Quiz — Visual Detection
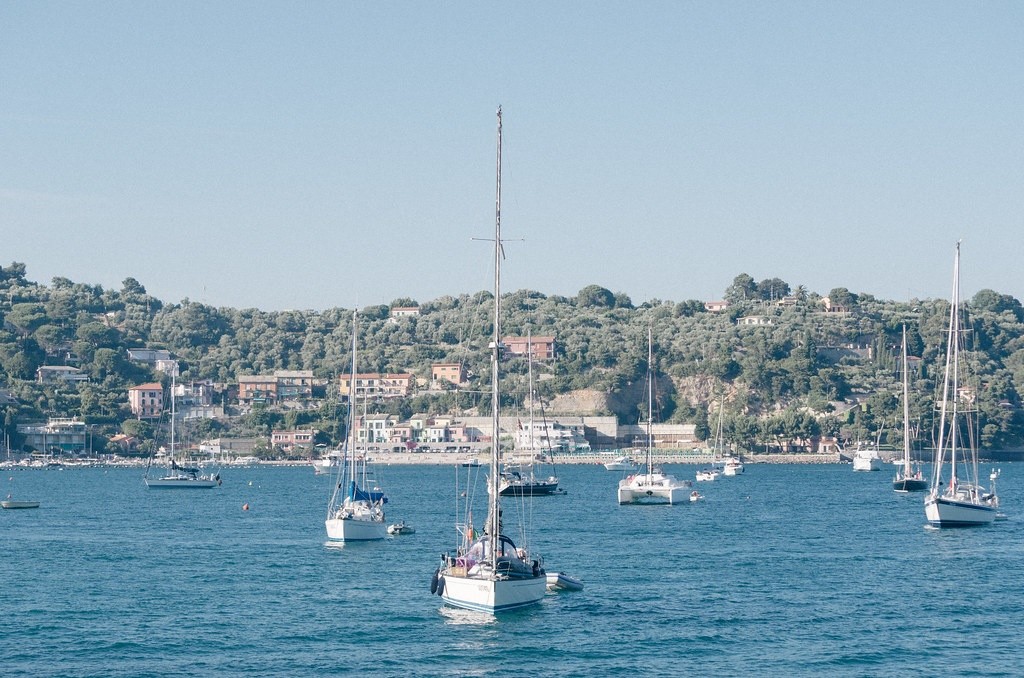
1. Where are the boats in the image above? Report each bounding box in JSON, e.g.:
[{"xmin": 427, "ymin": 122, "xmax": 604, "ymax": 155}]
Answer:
[
  {"xmin": 1, "ymin": 499, "xmax": 41, "ymax": 510},
  {"xmin": 546, "ymin": 570, "xmax": 585, "ymax": 594},
  {"xmin": 695, "ymin": 471, "xmax": 723, "ymax": 483},
  {"xmin": 724, "ymin": 455, "xmax": 744, "ymax": 477},
  {"xmin": 603, "ymin": 455, "xmax": 639, "ymax": 472},
  {"xmin": 852, "ymin": 449, "xmax": 884, "ymax": 472}
]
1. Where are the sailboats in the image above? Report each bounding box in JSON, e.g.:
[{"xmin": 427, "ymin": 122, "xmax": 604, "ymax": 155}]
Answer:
[
  {"xmin": 890, "ymin": 324, "xmax": 927, "ymax": 491},
  {"xmin": 312, "ymin": 307, "xmax": 419, "ymax": 542},
  {"xmin": 428, "ymin": 105, "xmax": 549, "ymax": 610},
  {"xmin": 923, "ymin": 239, "xmax": 998, "ymax": 526},
  {"xmin": 616, "ymin": 324, "xmax": 694, "ymax": 505},
  {"xmin": 498, "ymin": 322, "xmax": 560, "ymax": 497},
  {"xmin": 142, "ymin": 365, "xmax": 222, "ymax": 489}
]
